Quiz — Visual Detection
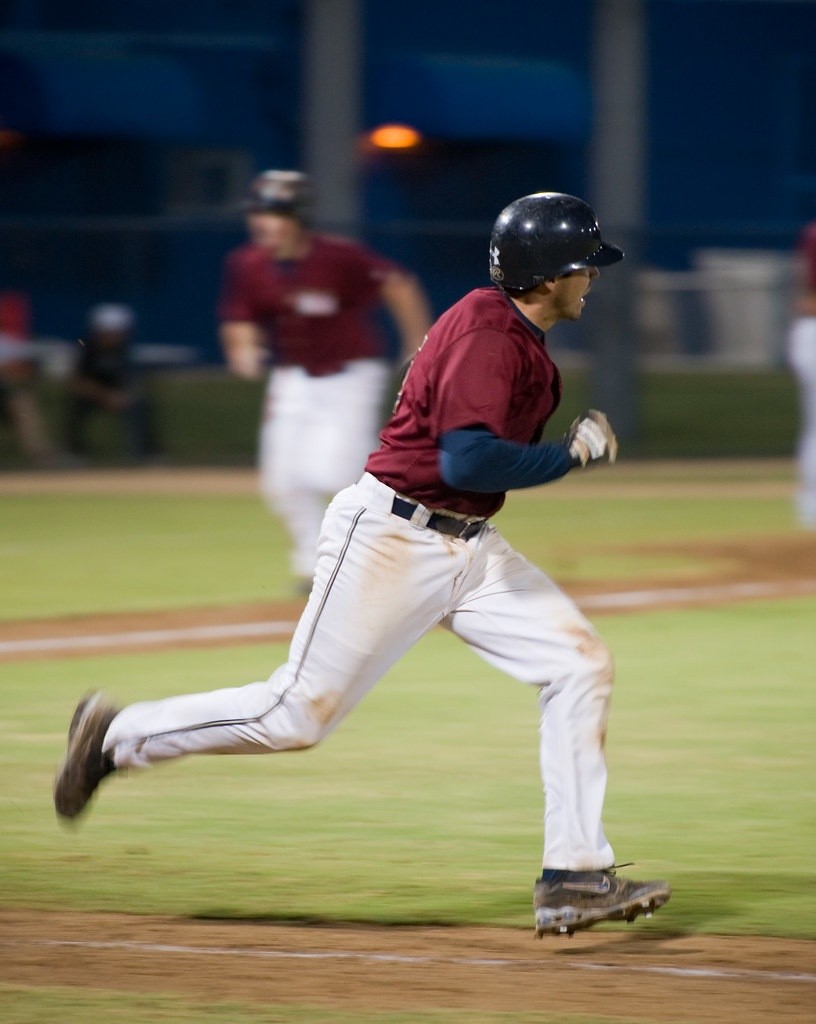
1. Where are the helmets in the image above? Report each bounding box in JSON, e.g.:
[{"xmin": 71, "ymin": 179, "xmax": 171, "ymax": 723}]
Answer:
[
  {"xmin": 231, "ymin": 170, "xmax": 322, "ymax": 226},
  {"xmin": 489, "ymin": 192, "xmax": 624, "ymax": 290}
]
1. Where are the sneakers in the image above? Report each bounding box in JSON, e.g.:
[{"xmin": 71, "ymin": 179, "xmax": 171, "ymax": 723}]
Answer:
[
  {"xmin": 54, "ymin": 689, "xmax": 120, "ymax": 814},
  {"xmin": 533, "ymin": 863, "xmax": 671, "ymax": 939}
]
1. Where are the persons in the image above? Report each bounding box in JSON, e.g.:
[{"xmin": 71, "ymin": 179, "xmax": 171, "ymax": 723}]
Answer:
[
  {"xmin": 58, "ymin": 302, "xmax": 158, "ymax": 464},
  {"xmin": 787, "ymin": 223, "xmax": 816, "ymax": 536},
  {"xmin": 220, "ymin": 170, "xmax": 428, "ymax": 598},
  {"xmin": 55, "ymin": 192, "xmax": 670, "ymax": 942},
  {"xmin": 0, "ymin": 293, "xmax": 56, "ymax": 467}
]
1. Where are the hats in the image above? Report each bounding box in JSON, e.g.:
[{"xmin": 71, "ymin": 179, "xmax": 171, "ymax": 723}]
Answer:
[{"xmin": 90, "ymin": 301, "xmax": 134, "ymax": 331}]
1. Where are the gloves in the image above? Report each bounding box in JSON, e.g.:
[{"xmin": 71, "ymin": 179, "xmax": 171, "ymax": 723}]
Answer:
[{"xmin": 563, "ymin": 409, "xmax": 619, "ymax": 469}]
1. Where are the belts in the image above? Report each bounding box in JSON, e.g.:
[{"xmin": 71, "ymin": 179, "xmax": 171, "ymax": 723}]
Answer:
[{"xmin": 391, "ymin": 496, "xmax": 489, "ymax": 539}]
